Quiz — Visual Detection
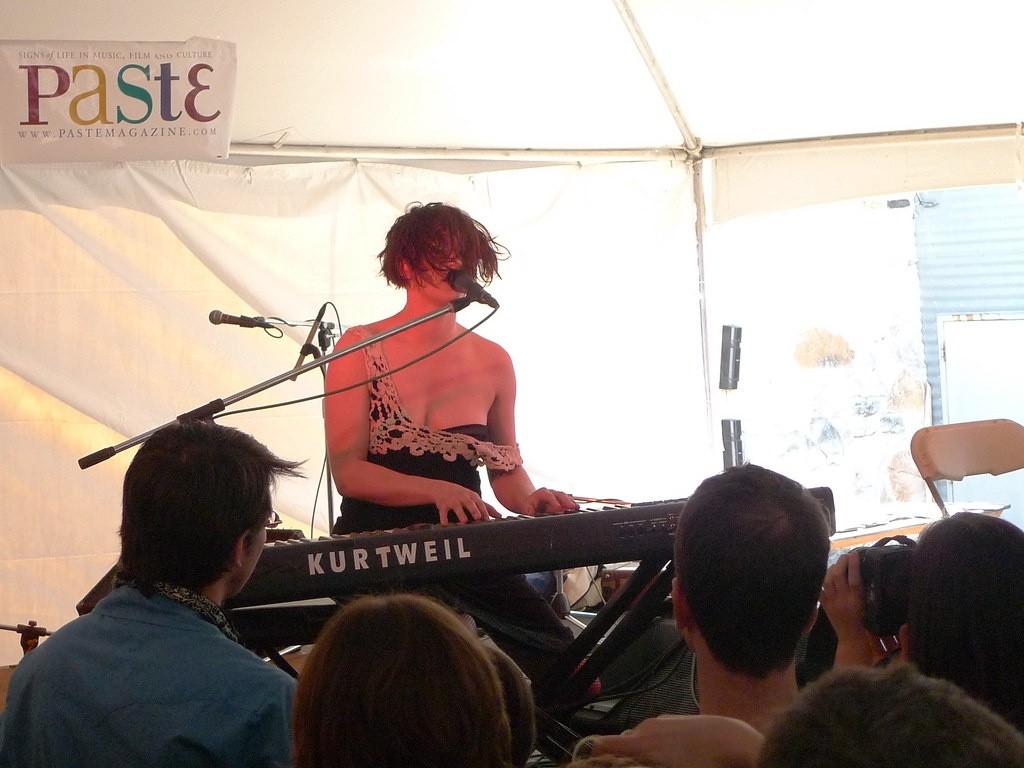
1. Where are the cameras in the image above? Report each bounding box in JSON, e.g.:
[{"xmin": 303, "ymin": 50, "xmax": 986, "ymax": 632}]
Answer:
[{"xmin": 847, "ymin": 545, "xmax": 915, "ymax": 637}]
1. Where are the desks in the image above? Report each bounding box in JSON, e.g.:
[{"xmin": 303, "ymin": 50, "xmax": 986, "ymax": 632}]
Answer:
[{"xmin": 830, "ymin": 501, "xmax": 1007, "ymax": 555}]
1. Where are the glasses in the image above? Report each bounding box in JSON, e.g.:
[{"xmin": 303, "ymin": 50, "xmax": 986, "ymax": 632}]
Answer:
[{"xmin": 260, "ymin": 511, "xmax": 282, "ymax": 528}]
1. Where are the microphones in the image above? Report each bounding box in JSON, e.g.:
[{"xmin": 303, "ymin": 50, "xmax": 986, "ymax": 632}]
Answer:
[
  {"xmin": 209, "ymin": 310, "xmax": 273, "ymax": 328},
  {"xmin": 291, "ymin": 304, "xmax": 327, "ymax": 381},
  {"xmin": 447, "ymin": 269, "xmax": 499, "ymax": 309}
]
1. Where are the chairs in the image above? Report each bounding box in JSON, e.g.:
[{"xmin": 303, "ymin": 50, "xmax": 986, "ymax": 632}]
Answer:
[{"xmin": 911, "ymin": 419, "xmax": 1024, "ymax": 518}]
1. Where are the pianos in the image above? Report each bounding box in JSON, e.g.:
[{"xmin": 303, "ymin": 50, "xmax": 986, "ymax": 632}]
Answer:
[{"xmin": 220, "ymin": 484, "xmax": 841, "ymax": 610}]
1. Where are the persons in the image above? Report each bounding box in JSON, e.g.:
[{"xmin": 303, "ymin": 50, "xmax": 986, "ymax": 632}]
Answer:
[
  {"xmin": 7, "ymin": 421, "xmax": 1024, "ymax": 767},
  {"xmin": 324, "ymin": 203, "xmax": 577, "ymax": 699}
]
